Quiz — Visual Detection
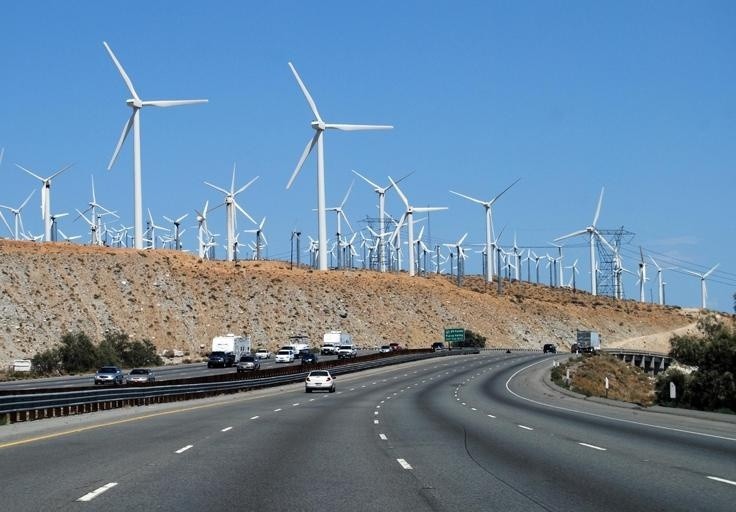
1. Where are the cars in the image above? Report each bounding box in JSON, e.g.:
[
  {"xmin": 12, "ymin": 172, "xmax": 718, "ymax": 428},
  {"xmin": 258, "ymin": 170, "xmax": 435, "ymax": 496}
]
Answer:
[
  {"xmin": 304, "ymin": 370, "xmax": 336, "ymax": 394},
  {"xmin": 125, "ymin": 368, "xmax": 154, "ymax": 384},
  {"xmin": 207, "ymin": 331, "xmax": 356, "ymax": 373},
  {"xmin": 543, "ymin": 344, "xmax": 556, "ymax": 353},
  {"xmin": 93, "ymin": 366, "xmax": 123, "ymax": 385},
  {"xmin": 431, "ymin": 342, "xmax": 443, "ymax": 351},
  {"xmin": 571, "ymin": 344, "xmax": 576, "ymax": 353},
  {"xmin": 379, "ymin": 343, "xmax": 401, "ymax": 354}
]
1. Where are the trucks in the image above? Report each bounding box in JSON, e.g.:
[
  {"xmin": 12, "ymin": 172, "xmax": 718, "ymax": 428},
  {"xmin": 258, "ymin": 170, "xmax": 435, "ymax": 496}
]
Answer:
[{"xmin": 576, "ymin": 330, "xmax": 601, "ymax": 352}]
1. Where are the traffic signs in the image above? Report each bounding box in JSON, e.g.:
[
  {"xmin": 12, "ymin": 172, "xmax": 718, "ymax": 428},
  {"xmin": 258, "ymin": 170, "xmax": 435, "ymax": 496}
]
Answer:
[{"xmin": 444, "ymin": 329, "xmax": 465, "ymax": 342}]
